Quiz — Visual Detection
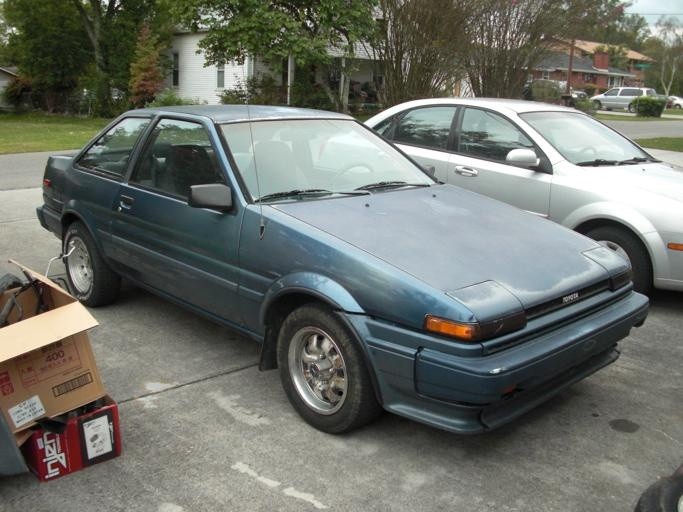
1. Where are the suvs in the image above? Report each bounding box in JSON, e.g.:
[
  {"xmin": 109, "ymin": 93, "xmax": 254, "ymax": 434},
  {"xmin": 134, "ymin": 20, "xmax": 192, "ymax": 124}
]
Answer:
[{"xmin": 522, "ymin": 77, "xmax": 587, "ymax": 105}]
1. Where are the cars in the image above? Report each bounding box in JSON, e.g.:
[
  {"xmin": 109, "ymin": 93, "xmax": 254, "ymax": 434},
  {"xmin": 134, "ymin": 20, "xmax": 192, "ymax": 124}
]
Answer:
[
  {"xmin": 111, "ymin": 88, "xmax": 123, "ymax": 103},
  {"xmin": 36, "ymin": 101, "xmax": 649, "ymax": 436},
  {"xmin": 316, "ymin": 95, "xmax": 682, "ymax": 293},
  {"xmin": 666, "ymin": 93, "xmax": 682, "ymax": 109}
]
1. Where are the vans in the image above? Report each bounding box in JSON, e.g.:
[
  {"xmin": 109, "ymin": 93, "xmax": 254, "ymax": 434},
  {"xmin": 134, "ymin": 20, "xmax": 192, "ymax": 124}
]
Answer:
[{"xmin": 587, "ymin": 86, "xmax": 657, "ymax": 113}]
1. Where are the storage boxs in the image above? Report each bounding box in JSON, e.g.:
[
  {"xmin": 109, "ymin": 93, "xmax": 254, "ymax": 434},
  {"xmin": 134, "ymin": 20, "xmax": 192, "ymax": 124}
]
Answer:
[
  {"xmin": 16, "ymin": 394, "xmax": 124, "ymax": 481},
  {"xmin": 0, "ymin": 256, "xmax": 107, "ymax": 439}
]
